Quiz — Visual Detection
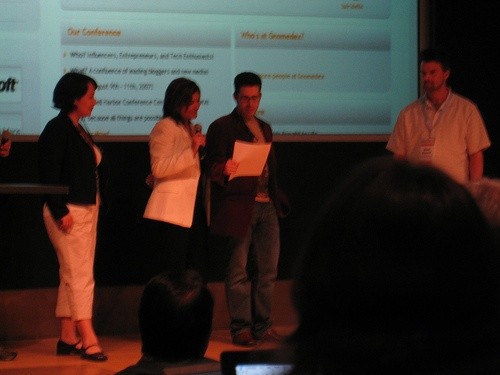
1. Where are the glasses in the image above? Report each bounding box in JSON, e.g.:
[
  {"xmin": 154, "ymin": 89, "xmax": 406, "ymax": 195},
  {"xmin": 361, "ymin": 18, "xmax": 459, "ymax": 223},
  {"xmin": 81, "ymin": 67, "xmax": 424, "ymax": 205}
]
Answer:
[{"xmin": 237, "ymin": 93, "xmax": 261, "ymax": 102}]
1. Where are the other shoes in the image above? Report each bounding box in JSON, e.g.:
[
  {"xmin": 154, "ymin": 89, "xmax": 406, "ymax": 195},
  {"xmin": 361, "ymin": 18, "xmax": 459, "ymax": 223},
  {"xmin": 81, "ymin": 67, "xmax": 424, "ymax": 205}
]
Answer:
[
  {"xmin": 57, "ymin": 338, "xmax": 83, "ymax": 356},
  {"xmin": 232, "ymin": 330, "xmax": 256, "ymax": 347},
  {"xmin": 253, "ymin": 328, "xmax": 284, "ymax": 343},
  {"xmin": 80, "ymin": 344, "xmax": 108, "ymax": 362}
]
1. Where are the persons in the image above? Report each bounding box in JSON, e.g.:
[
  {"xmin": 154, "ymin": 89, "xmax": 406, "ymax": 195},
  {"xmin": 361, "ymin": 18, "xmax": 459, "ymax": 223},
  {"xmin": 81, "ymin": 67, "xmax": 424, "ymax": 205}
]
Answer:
[
  {"xmin": 388, "ymin": 51, "xmax": 492, "ymax": 181},
  {"xmin": 0, "ymin": 127, "xmax": 20, "ymax": 361},
  {"xmin": 206, "ymin": 73, "xmax": 287, "ymax": 348},
  {"xmin": 288, "ymin": 156, "xmax": 500, "ymax": 374},
  {"xmin": 143, "ymin": 77, "xmax": 207, "ymax": 281},
  {"xmin": 38, "ymin": 73, "xmax": 108, "ymax": 362},
  {"xmin": 115, "ymin": 270, "xmax": 221, "ymax": 375}
]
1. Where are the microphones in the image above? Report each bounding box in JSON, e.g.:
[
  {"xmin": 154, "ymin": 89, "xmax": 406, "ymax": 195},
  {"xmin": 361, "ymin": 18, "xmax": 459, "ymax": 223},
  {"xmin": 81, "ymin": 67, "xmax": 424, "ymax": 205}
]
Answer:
[{"xmin": 193, "ymin": 123, "xmax": 204, "ymax": 160}]
